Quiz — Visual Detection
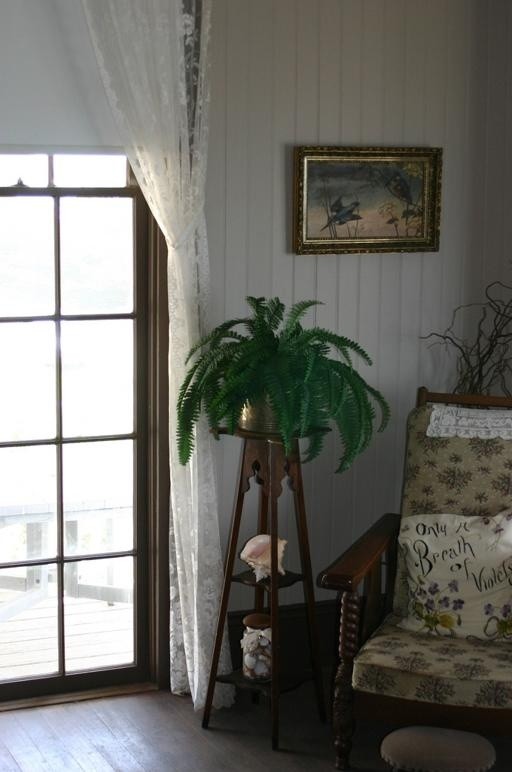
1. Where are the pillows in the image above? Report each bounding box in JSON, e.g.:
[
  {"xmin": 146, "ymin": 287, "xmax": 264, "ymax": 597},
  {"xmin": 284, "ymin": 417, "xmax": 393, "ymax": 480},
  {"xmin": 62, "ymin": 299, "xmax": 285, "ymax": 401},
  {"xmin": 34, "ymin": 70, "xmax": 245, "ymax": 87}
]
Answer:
[{"xmin": 397, "ymin": 512, "xmax": 511, "ymax": 637}]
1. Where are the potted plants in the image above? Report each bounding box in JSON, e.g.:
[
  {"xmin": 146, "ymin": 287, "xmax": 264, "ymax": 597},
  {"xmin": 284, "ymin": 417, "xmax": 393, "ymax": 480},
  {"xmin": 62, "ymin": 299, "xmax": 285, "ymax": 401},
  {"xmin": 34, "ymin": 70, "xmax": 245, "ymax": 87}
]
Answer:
[{"xmin": 177, "ymin": 295, "xmax": 390, "ymax": 475}]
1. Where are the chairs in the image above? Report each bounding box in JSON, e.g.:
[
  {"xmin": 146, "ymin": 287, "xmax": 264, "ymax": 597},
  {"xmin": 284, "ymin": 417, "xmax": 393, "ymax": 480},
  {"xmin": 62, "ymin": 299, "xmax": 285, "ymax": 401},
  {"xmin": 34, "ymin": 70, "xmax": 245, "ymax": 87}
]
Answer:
[{"xmin": 315, "ymin": 386, "xmax": 512, "ymax": 772}]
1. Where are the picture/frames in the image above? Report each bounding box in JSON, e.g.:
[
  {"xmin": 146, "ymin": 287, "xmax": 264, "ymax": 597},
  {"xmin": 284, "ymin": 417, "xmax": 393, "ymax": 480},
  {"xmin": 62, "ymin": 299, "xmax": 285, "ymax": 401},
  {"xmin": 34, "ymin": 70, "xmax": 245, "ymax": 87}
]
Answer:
[{"xmin": 292, "ymin": 142, "xmax": 442, "ymax": 256}]
